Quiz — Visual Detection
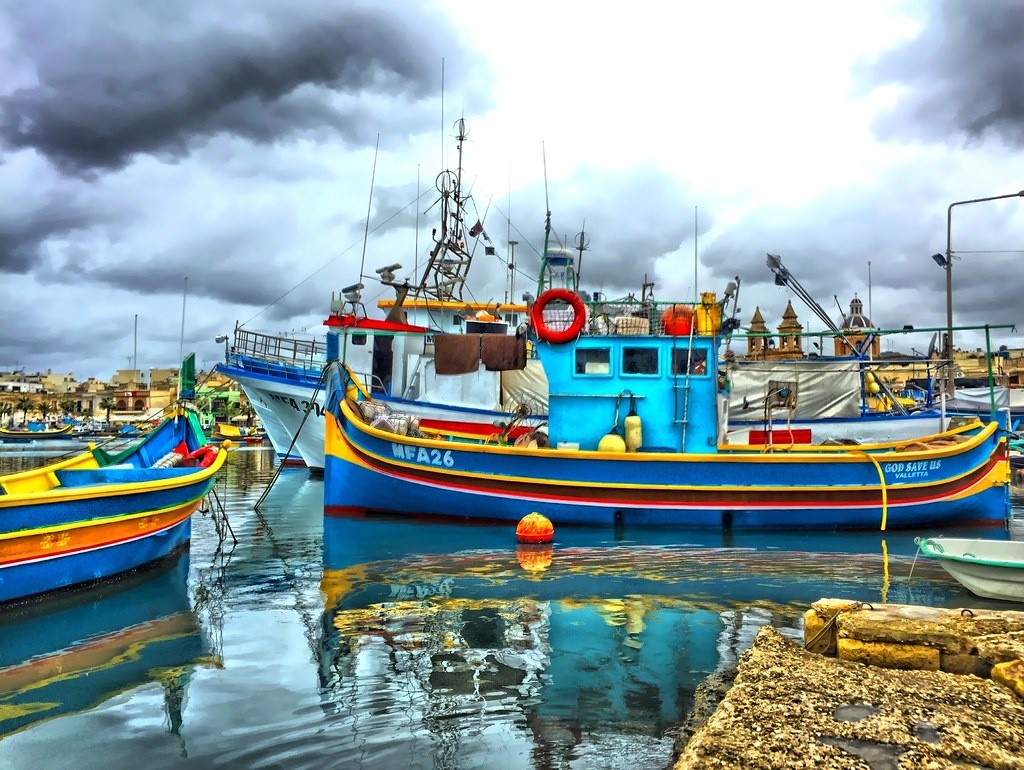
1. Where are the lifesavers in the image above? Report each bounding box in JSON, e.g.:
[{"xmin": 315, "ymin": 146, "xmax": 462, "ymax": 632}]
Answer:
[
  {"xmin": 531, "ymin": 289, "xmax": 585, "ymax": 343},
  {"xmin": 188, "ymin": 445, "xmax": 219, "ymax": 468}
]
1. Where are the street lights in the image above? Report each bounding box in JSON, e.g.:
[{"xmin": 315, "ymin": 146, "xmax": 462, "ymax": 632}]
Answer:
[{"xmin": 931, "ymin": 252, "xmax": 955, "ymax": 400}]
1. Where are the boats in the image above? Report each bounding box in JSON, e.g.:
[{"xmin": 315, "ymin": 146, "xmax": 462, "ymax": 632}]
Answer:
[
  {"xmin": 0, "ymin": 351, "xmax": 233, "ymax": 603},
  {"xmin": 79, "ymin": 436, "xmax": 117, "ymax": 442},
  {"xmin": 0, "ymin": 423, "xmax": 74, "ymax": 439},
  {"xmin": 323, "ymin": 116, "xmax": 1012, "ymax": 525},
  {"xmin": 213, "ymin": 55, "xmax": 524, "ymax": 481}
]
